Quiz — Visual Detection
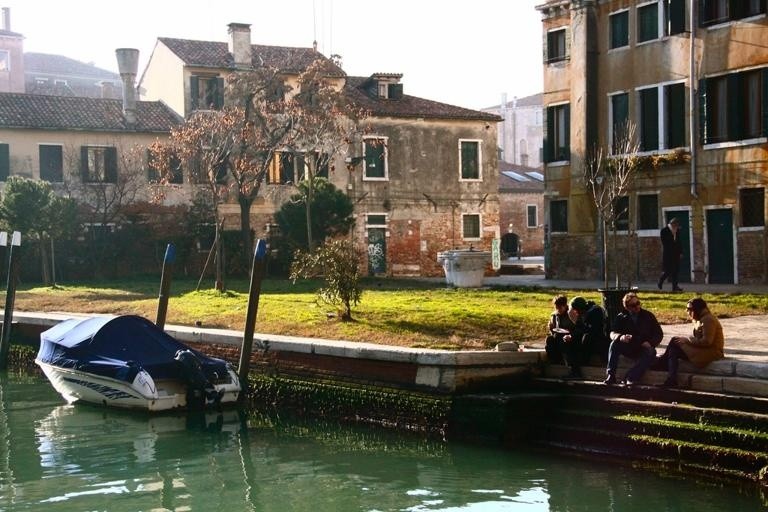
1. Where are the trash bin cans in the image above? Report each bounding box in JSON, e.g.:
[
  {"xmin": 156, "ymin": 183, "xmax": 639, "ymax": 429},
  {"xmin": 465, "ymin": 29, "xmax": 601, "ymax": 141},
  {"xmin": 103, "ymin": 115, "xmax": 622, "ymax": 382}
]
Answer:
[{"xmin": 598, "ymin": 286, "xmax": 639, "ymax": 327}]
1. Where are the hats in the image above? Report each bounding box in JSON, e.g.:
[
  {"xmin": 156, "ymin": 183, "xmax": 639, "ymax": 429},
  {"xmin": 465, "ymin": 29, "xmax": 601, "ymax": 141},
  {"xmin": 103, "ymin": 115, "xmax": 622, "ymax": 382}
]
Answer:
[{"xmin": 570, "ymin": 296, "xmax": 586, "ymax": 310}]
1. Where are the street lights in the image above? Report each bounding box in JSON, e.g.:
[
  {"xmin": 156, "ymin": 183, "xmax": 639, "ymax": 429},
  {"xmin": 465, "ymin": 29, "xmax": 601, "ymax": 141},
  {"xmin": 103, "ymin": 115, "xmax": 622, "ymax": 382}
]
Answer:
[
  {"xmin": 595, "ymin": 176, "xmax": 606, "ymax": 282},
  {"xmin": 348, "ymin": 155, "xmax": 375, "ymax": 257}
]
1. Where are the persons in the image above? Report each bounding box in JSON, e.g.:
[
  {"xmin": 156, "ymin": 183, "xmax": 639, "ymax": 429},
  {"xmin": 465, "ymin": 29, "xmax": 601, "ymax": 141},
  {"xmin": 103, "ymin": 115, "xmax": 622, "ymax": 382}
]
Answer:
[
  {"xmin": 658, "ymin": 217, "xmax": 684, "ymax": 291},
  {"xmin": 545, "ymin": 293, "xmax": 724, "ymax": 388}
]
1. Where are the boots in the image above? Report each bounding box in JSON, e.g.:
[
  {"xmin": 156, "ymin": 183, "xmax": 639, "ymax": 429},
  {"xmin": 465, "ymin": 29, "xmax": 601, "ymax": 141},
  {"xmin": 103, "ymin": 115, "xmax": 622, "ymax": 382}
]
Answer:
[{"xmin": 655, "ymin": 378, "xmax": 678, "ymax": 389}]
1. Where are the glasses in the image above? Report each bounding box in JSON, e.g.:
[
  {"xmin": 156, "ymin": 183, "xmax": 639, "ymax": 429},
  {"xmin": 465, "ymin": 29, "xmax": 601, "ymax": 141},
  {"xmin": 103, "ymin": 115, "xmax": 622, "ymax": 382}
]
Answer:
[
  {"xmin": 627, "ymin": 301, "xmax": 640, "ymax": 307},
  {"xmin": 687, "ymin": 308, "xmax": 695, "ymax": 310}
]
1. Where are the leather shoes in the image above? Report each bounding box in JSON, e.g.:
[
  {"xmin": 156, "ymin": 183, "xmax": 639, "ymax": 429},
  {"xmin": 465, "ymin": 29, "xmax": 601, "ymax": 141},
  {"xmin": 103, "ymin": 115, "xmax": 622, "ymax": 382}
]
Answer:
[
  {"xmin": 621, "ymin": 377, "xmax": 634, "ymax": 385},
  {"xmin": 602, "ymin": 374, "xmax": 616, "ymax": 383}
]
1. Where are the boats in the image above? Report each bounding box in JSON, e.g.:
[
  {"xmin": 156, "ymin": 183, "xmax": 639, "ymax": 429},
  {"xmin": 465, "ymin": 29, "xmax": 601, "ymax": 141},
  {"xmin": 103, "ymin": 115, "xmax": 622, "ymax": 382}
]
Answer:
[
  {"xmin": 35, "ymin": 404, "xmax": 240, "ymax": 478},
  {"xmin": 34, "ymin": 315, "xmax": 241, "ymax": 413}
]
